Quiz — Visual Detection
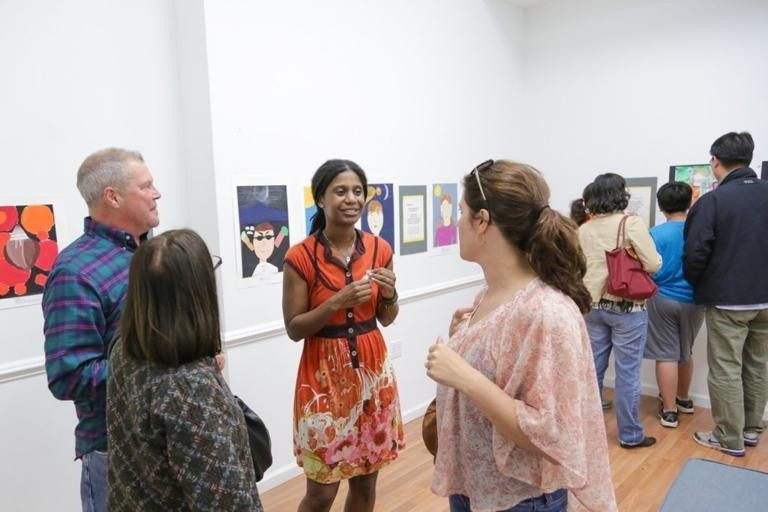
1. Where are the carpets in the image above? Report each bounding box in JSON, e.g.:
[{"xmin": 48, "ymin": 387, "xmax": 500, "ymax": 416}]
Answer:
[{"xmin": 656, "ymin": 457, "xmax": 767, "ymax": 512}]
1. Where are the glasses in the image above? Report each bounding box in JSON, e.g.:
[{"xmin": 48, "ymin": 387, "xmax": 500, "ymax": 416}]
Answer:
[
  {"xmin": 207, "ymin": 257, "xmax": 222, "ymax": 274},
  {"xmin": 473, "ymin": 159, "xmax": 493, "ymax": 209},
  {"xmin": 253, "ymin": 235, "xmax": 274, "ymax": 240}
]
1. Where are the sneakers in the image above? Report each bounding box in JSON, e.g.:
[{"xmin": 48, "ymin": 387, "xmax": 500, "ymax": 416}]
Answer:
[
  {"xmin": 658, "ymin": 393, "xmax": 694, "ymax": 414},
  {"xmin": 658, "ymin": 406, "xmax": 679, "ymax": 428},
  {"xmin": 601, "ymin": 399, "xmax": 612, "ymax": 409},
  {"xmin": 693, "ymin": 431, "xmax": 746, "ymax": 457},
  {"xmin": 744, "ymin": 431, "xmax": 762, "ymax": 447}
]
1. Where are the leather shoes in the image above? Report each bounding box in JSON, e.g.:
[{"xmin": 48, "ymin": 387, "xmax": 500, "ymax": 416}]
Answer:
[{"xmin": 620, "ymin": 436, "xmax": 655, "ymax": 449}]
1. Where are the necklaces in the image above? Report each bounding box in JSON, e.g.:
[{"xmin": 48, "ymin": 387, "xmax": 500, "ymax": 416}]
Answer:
[{"xmin": 322, "ymin": 231, "xmax": 357, "ymax": 270}]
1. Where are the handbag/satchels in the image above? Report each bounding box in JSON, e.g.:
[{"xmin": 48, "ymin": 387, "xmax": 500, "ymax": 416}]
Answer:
[
  {"xmin": 604, "ymin": 214, "xmax": 658, "ymax": 300},
  {"xmin": 234, "ymin": 395, "xmax": 272, "ymax": 483},
  {"xmin": 422, "ymin": 398, "xmax": 437, "ymax": 458}
]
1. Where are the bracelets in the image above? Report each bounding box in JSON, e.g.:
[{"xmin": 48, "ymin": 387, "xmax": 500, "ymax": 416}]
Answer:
[{"xmin": 378, "ymin": 291, "xmax": 397, "ymax": 310}]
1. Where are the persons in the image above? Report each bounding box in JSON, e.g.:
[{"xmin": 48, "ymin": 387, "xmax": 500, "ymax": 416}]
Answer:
[
  {"xmin": 570, "ymin": 183, "xmax": 598, "ymax": 225},
  {"xmin": 41, "ymin": 146, "xmax": 161, "ymax": 512},
  {"xmin": 424, "ymin": 159, "xmax": 617, "ymax": 512},
  {"xmin": 106, "ymin": 229, "xmax": 265, "ymax": 512},
  {"xmin": 435, "ymin": 194, "xmax": 457, "ymax": 248},
  {"xmin": 241, "ymin": 222, "xmax": 289, "ymax": 280},
  {"xmin": 682, "ymin": 132, "xmax": 768, "ymax": 457},
  {"xmin": 283, "ymin": 159, "xmax": 400, "ymax": 512},
  {"xmin": 644, "ymin": 180, "xmax": 705, "ymax": 429},
  {"xmin": 366, "ymin": 199, "xmax": 392, "ymax": 252},
  {"xmin": 575, "ymin": 174, "xmax": 657, "ymax": 449}
]
